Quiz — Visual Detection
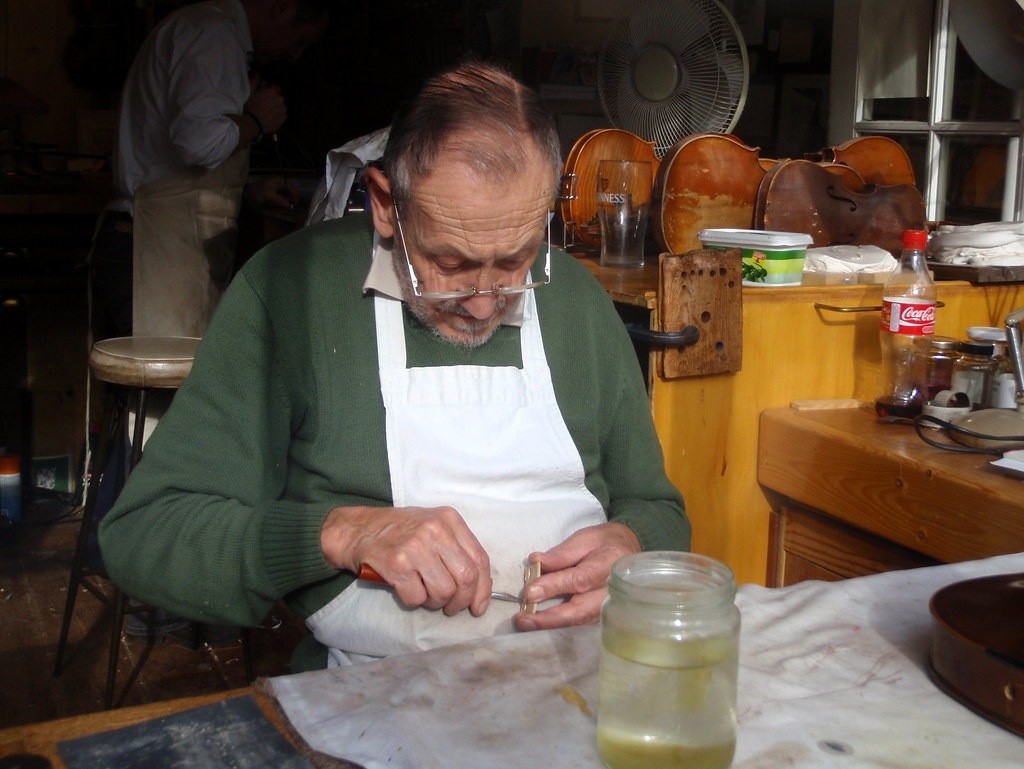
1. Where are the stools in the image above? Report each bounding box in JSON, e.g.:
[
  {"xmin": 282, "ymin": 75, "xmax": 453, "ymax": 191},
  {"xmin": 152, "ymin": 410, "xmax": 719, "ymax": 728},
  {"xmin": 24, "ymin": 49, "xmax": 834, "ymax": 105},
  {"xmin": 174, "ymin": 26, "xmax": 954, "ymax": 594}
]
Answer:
[{"xmin": 51, "ymin": 335, "xmax": 254, "ymax": 710}]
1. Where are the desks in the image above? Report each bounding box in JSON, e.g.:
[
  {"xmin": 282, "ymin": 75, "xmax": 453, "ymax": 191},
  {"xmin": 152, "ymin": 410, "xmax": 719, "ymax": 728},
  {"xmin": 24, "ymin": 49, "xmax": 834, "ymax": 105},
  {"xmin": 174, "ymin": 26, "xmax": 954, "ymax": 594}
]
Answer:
[
  {"xmin": 0, "ymin": 548, "xmax": 1023, "ymax": 769},
  {"xmin": 546, "ymin": 239, "xmax": 1023, "ymax": 588},
  {"xmin": 755, "ymin": 402, "xmax": 1024, "ymax": 591}
]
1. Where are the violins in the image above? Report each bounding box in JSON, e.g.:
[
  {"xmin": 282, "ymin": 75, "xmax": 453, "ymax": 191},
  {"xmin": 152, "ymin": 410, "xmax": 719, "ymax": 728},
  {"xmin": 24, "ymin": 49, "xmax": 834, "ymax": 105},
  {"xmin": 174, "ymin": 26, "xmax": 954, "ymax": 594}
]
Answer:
[
  {"xmin": 555, "ymin": 113, "xmax": 928, "ymax": 267},
  {"xmin": 920, "ymin": 570, "xmax": 1024, "ymax": 739}
]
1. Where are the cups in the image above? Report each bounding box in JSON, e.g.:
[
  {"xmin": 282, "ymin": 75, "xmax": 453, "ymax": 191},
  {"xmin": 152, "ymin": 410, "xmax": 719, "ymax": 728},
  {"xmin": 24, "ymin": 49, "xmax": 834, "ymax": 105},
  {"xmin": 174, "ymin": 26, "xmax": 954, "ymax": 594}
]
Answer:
[{"xmin": 596, "ymin": 159, "xmax": 654, "ymax": 269}]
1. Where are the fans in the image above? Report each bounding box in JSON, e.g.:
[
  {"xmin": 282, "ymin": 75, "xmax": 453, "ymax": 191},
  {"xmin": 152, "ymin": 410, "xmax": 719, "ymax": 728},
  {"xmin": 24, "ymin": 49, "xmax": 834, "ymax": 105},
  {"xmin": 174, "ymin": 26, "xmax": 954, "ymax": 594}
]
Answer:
[{"xmin": 595, "ymin": 0, "xmax": 750, "ymax": 159}]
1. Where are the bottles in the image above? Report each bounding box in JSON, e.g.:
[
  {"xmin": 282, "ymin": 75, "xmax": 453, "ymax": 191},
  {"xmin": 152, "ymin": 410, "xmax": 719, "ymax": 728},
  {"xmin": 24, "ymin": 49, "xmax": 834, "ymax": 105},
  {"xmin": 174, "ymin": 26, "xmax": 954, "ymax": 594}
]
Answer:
[
  {"xmin": 0, "ymin": 454, "xmax": 22, "ymax": 528},
  {"xmin": 926, "ymin": 326, "xmax": 1018, "ymax": 411},
  {"xmin": 595, "ymin": 550, "xmax": 741, "ymax": 769},
  {"xmin": 874, "ymin": 229, "xmax": 938, "ymax": 421}
]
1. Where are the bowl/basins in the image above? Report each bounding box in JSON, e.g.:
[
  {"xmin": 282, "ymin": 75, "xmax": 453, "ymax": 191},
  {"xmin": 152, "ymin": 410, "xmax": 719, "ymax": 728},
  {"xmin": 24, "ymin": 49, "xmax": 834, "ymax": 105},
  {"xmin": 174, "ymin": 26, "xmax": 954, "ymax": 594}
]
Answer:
[{"xmin": 698, "ymin": 228, "xmax": 815, "ymax": 287}]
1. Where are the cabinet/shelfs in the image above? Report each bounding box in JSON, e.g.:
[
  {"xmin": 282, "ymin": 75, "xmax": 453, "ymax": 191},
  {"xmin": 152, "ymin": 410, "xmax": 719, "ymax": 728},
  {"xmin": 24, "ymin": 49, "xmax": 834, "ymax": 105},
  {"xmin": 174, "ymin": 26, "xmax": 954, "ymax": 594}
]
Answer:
[{"xmin": 851, "ymin": 1, "xmax": 1024, "ymax": 233}]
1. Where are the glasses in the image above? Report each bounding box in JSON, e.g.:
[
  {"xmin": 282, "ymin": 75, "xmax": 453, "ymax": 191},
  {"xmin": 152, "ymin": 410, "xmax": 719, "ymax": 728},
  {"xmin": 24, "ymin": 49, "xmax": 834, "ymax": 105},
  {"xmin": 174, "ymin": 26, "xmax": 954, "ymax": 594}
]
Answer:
[{"xmin": 380, "ymin": 169, "xmax": 552, "ymax": 301}]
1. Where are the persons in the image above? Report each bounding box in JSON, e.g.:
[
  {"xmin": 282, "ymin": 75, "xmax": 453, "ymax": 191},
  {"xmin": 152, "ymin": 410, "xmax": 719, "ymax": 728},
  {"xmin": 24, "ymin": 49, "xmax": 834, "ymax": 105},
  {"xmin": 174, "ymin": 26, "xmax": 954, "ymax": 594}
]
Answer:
[
  {"xmin": 97, "ymin": 57, "xmax": 694, "ymax": 683},
  {"xmin": 88, "ymin": 0, "xmax": 332, "ymax": 635}
]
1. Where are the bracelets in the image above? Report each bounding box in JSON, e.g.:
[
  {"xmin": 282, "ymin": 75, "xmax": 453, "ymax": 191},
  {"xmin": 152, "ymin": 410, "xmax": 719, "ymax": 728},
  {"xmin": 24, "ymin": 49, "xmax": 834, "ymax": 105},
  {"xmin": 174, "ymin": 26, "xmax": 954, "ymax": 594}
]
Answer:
[{"xmin": 242, "ymin": 113, "xmax": 263, "ymax": 146}]
